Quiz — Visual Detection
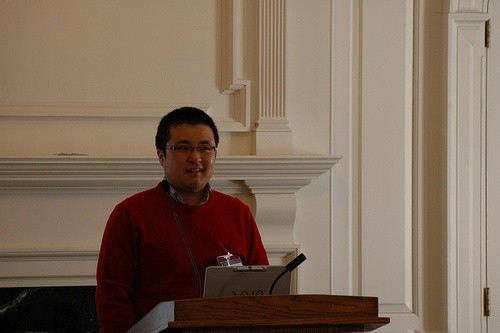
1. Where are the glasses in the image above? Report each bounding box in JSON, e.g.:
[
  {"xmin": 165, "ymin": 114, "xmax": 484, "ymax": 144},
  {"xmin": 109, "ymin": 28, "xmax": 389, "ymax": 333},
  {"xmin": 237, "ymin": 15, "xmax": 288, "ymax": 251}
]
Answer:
[{"xmin": 164, "ymin": 144, "xmax": 216, "ymax": 155}]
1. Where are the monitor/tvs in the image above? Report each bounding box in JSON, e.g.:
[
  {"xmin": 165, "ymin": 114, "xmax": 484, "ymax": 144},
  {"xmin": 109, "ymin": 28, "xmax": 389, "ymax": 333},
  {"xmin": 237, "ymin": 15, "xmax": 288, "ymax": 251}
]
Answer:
[{"xmin": 204, "ymin": 266, "xmax": 291, "ymax": 297}]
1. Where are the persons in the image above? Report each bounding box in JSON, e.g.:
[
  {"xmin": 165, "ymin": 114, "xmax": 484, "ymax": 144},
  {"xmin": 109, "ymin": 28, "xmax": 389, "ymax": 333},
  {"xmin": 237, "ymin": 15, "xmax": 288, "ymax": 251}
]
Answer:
[{"xmin": 96, "ymin": 107, "xmax": 269, "ymax": 333}]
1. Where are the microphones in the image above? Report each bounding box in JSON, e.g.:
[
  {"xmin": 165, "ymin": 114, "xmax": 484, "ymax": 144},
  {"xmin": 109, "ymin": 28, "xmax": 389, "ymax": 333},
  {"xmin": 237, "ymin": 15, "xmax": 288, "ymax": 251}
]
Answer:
[
  {"xmin": 162, "ymin": 180, "xmax": 201, "ymax": 299},
  {"xmin": 269, "ymin": 253, "xmax": 307, "ymax": 295}
]
